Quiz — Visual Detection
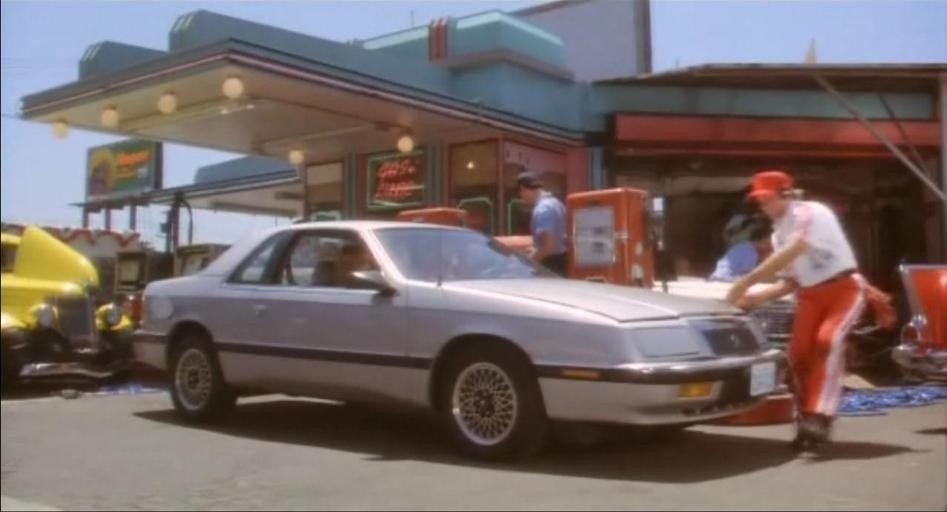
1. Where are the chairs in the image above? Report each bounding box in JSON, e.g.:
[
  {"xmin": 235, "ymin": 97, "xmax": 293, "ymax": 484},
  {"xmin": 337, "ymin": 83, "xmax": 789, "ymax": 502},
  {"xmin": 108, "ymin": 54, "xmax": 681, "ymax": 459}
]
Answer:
[{"xmin": 310, "ymin": 261, "xmax": 336, "ymax": 286}]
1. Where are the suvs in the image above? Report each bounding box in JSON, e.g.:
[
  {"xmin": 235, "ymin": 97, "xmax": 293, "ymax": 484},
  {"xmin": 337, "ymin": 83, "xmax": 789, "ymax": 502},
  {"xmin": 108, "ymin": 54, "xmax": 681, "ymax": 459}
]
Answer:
[{"xmin": 0, "ymin": 224, "xmax": 139, "ymax": 396}]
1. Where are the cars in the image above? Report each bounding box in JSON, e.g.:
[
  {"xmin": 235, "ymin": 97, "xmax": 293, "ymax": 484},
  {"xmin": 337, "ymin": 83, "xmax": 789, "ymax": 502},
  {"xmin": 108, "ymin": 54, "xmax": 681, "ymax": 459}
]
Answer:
[
  {"xmin": 649, "ymin": 275, "xmax": 796, "ymax": 372},
  {"xmin": 127, "ymin": 215, "xmax": 789, "ymax": 462}
]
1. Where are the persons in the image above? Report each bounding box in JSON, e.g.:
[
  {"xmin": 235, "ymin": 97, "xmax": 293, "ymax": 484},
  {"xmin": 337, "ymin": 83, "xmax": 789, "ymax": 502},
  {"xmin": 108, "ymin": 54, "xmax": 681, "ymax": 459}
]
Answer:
[
  {"xmin": 726, "ymin": 171, "xmax": 867, "ymax": 457},
  {"xmin": 721, "ymin": 188, "xmax": 767, "ymax": 240},
  {"xmin": 708, "ymin": 227, "xmax": 775, "ymax": 281},
  {"xmin": 517, "ymin": 171, "xmax": 567, "ymax": 277}
]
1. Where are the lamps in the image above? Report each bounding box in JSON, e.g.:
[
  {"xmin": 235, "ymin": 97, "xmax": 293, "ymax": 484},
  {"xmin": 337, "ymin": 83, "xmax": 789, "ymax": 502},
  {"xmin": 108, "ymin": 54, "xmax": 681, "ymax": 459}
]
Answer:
[
  {"xmin": 157, "ymin": 92, "xmax": 176, "ymax": 115},
  {"xmin": 52, "ymin": 120, "xmax": 66, "ymax": 136},
  {"xmin": 221, "ymin": 77, "xmax": 243, "ymax": 100},
  {"xmin": 396, "ymin": 136, "xmax": 413, "ymax": 155},
  {"xmin": 290, "ymin": 148, "xmax": 305, "ymax": 165},
  {"xmin": 98, "ymin": 107, "xmax": 119, "ymax": 129}
]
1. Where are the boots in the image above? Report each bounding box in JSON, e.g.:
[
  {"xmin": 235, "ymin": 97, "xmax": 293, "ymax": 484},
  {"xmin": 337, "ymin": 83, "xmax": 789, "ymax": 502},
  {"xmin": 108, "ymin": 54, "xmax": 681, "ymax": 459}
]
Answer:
[{"xmin": 783, "ymin": 413, "xmax": 832, "ymax": 459}]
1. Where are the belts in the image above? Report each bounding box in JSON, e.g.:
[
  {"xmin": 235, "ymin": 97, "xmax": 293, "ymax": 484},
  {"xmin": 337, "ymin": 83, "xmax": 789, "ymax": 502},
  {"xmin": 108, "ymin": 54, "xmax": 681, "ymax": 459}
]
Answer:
[{"xmin": 819, "ymin": 269, "xmax": 860, "ymax": 285}]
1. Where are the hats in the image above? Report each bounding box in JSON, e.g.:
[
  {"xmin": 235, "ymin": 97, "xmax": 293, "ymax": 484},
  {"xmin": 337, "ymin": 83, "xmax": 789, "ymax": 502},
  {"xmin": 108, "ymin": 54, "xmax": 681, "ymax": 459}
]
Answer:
[
  {"xmin": 748, "ymin": 171, "xmax": 792, "ymax": 199},
  {"xmin": 517, "ymin": 172, "xmax": 544, "ymax": 187}
]
